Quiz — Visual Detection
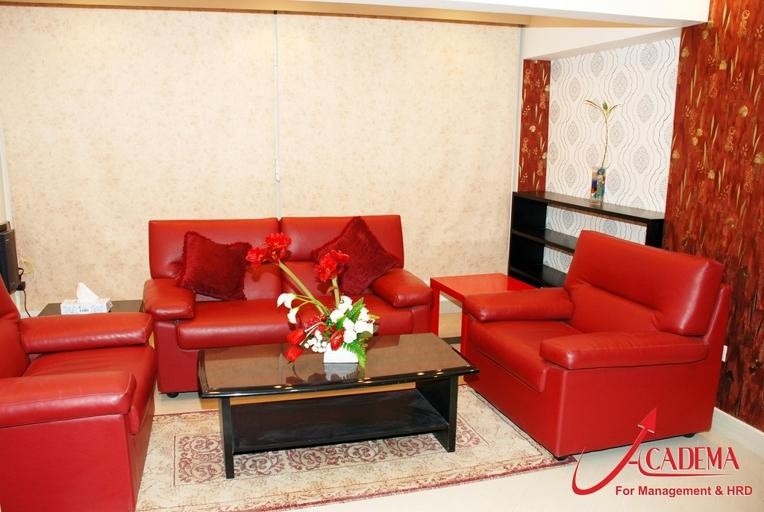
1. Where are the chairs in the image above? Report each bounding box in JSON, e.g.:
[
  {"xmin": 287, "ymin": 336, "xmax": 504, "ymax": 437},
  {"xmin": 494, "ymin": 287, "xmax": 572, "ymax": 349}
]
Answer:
[
  {"xmin": 0, "ymin": 275, "xmax": 160, "ymax": 512},
  {"xmin": 463, "ymin": 228, "xmax": 735, "ymax": 461}
]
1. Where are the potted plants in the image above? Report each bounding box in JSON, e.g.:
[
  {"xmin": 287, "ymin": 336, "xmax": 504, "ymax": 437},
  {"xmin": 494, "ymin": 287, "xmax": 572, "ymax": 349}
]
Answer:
[{"xmin": 584, "ymin": 95, "xmax": 621, "ymax": 203}]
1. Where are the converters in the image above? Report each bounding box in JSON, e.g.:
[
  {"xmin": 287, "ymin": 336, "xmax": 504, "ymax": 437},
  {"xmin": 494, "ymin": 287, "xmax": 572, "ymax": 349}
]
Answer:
[{"xmin": 16, "ymin": 281, "xmax": 25, "ymax": 291}]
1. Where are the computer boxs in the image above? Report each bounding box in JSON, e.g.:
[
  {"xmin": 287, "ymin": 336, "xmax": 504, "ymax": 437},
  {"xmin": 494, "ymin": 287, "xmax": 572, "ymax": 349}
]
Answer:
[{"xmin": 0, "ymin": 222, "xmax": 20, "ymax": 295}]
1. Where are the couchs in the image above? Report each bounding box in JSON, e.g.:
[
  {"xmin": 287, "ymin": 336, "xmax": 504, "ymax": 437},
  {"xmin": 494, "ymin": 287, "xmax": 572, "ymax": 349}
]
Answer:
[{"xmin": 143, "ymin": 214, "xmax": 434, "ymax": 400}]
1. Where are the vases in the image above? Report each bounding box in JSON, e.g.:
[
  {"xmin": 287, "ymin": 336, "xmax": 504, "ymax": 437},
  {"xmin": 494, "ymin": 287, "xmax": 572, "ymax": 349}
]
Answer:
[{"xmin": 321, "ymin": 349, "xmax": 360, "ymax": 381}]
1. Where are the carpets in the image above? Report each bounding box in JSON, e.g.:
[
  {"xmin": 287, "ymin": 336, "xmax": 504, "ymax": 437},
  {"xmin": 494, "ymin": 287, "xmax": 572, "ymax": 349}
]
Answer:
[{"xmin": 131, "ymin": 385, "xmax": 579, "ymax": 512}]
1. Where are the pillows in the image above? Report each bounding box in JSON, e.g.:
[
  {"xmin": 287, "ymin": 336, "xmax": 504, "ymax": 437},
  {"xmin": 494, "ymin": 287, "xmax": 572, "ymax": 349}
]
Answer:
[
  {"xmin": 309, "ymin": 215, "xmax": 402, "ymax": 302},
  {"xmin": 177, "ymin": 228, "xmax": 252, "ymax": 306}
]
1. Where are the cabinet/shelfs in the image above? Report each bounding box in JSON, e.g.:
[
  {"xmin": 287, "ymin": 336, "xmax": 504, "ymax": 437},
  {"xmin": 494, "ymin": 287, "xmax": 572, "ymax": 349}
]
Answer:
[{"xmin": 506, "ymin": 189, "xmax": 667, "ymax": 301}]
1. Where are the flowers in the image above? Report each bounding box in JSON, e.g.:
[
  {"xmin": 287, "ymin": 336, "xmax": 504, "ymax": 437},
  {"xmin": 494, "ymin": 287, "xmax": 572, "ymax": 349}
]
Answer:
[{"xmin": 244, "ymin": 231, "xmax": 384, "ymax": 383}]
